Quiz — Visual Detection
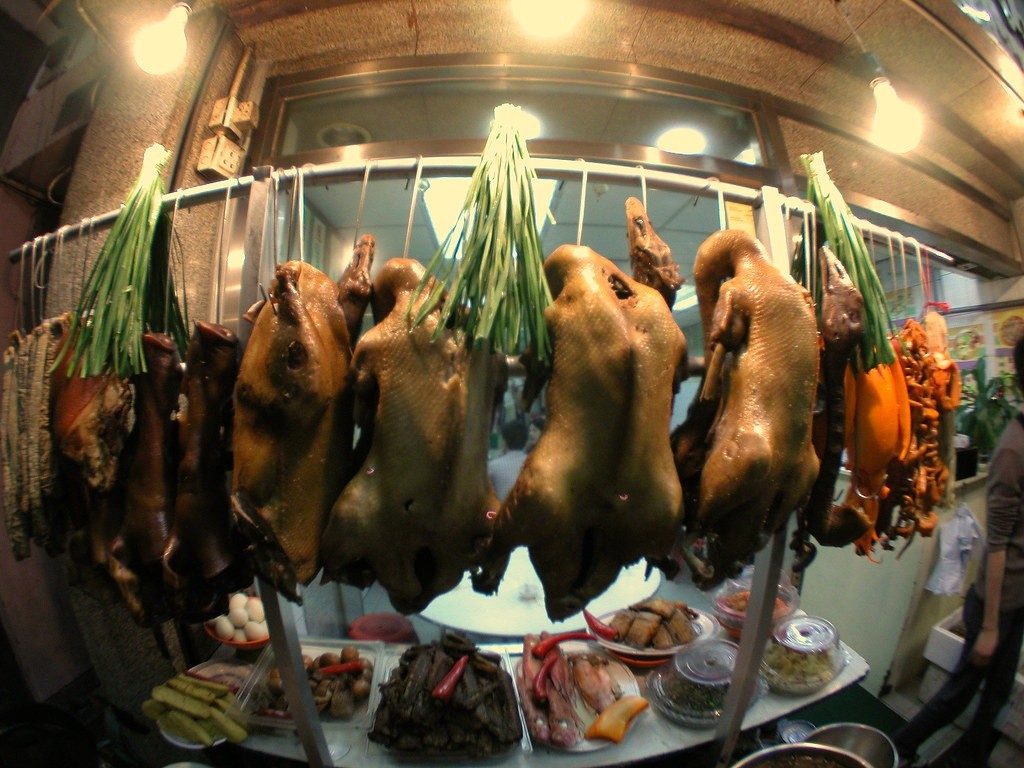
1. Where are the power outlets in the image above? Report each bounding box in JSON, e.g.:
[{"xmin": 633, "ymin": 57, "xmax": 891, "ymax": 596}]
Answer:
[{"xmin": 198, "ymin": 136, "xmax": 244, "ymax": 180}]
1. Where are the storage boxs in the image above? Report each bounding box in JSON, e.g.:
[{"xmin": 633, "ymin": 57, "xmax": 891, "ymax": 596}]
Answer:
[
  {"xmin": 922, "ymin": 605, "xmax": 966, "ymax": 673},
  {"xmin": 228, "ymin": 635, "xmax": 383, "ymax": 733}
]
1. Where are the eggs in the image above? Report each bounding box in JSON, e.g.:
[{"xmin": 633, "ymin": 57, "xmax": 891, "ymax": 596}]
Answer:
[{"xmin": 205, "ymin": 592, "xmax": 271, "ymax": 642}]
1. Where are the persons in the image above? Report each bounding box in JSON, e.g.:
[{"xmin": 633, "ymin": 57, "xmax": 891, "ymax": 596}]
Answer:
[{"xmin": 895, "ymin": 339, "xmax": 1024, "ymax": 768}]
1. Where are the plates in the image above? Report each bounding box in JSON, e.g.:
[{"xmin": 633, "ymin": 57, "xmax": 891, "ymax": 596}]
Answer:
[
  {"xmin": 517, "ymin": 641, "xmax": 641, "ymax": 753},
  {"xmin": 158, "ymin": 660, "xmax": 255, "ymax": 749},
  {"xmin": 586, "ymin": 609, "xmax": 720, "ymax": 656}
]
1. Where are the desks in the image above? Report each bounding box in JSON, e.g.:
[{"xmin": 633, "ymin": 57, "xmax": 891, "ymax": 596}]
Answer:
[{"xmin": 417, "ymin": 545, "xmax": 663, "ymax": 646}]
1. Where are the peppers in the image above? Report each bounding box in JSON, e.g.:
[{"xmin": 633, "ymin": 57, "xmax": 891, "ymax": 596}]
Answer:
[
  {"xmin": 534, "ymin": 654, "xmax": 559, "ymax": 705},
  {"xmin": 316, "ymin": 660, "xmax": 363, "ymax": 676},
  {"xmin": 431, "ymin": 654, "xmax": 469, "ymax": 707},
  {"xmin": 531, "ymin": 632, "xmax": 597, "ymax": 660},
  {"xmin": 251, "ymin": 707, "xmax": 293, "ymax": 720},
  {"xmin": 582, "ymin": 608, "xmax": 620, "ymax": 641}
]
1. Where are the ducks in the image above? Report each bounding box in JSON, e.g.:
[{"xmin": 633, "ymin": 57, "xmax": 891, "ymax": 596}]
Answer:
[{"xmin": 57, "ymin": 196, "xmax": 914, "ymax": 624}]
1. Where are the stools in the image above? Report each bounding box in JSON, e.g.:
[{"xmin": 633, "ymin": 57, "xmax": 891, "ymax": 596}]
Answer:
[{"xmin": 345, "ymin": 612, "xmax": 422, "ymax": 645}]
